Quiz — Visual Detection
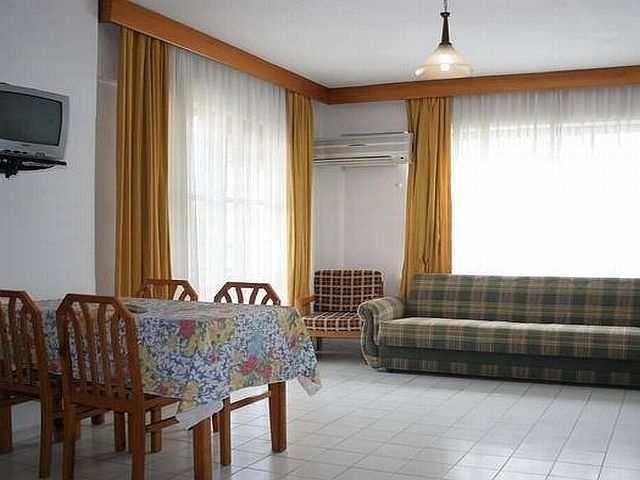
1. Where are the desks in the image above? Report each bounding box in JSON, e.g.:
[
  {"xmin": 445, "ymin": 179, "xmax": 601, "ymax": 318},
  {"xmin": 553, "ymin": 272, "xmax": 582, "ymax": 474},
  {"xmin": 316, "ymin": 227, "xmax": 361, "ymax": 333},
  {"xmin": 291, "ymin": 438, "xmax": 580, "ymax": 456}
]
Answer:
[{"xmin": 0, "ymin": 293, "xmax": 302, "ymax": 480}]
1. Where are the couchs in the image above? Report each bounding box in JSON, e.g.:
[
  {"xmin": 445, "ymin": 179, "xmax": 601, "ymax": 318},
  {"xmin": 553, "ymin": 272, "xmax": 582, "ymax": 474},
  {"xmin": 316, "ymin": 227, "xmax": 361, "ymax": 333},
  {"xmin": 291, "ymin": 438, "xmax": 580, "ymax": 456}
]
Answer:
[{"xmin": 353, "ymin": 271, "xmax": 640, "ymax": 390}]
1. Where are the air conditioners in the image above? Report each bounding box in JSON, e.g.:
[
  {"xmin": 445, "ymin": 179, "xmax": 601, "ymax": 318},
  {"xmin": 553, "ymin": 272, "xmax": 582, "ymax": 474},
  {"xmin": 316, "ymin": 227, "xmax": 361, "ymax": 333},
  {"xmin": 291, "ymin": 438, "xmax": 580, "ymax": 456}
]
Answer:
[{"xmin": 312, "ymin": 134, "xmax": 411, "ymax": 168}]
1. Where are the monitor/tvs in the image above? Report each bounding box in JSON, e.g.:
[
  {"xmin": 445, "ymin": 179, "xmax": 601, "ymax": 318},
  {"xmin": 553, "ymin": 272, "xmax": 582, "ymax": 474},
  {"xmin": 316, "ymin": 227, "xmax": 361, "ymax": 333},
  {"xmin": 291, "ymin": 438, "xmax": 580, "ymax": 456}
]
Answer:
[{"xmin": 0, "ymin": 83, "xmax": 70, "ymax": 167}]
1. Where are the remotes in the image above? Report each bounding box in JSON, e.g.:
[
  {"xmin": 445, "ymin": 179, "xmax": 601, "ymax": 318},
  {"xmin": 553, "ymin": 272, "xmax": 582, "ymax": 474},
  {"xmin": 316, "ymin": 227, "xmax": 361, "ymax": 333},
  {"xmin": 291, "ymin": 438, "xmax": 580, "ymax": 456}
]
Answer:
[{"xmin": 124, "ymin": 304, "xmax": 148, "ymax": 313}]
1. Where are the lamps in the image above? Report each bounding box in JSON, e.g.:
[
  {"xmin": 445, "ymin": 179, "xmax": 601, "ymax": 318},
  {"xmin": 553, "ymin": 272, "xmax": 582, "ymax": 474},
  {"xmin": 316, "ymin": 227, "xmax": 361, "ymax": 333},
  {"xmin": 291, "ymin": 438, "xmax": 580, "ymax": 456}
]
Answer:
[{"xmin": 412, "ymin": 0, "xmax": 476, "ymax": 84}]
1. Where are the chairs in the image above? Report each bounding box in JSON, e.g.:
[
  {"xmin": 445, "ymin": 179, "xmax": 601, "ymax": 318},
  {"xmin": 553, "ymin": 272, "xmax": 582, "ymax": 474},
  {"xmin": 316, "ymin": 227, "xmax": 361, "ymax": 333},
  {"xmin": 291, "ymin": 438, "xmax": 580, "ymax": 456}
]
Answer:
[
  {"xmin": 132, "ymin": 280, "xmax": 200, "ymax": 304},
  {"xmin": 0, "ymin": 289, "xmax": 126, "ymax": 480},
  {"xmin": 213, "ymin": 280, "xmax": 282, "ymax": 306},
  {"xmin": 55, "ymin": 291, "xmax": 182, "ymax": 480},
  {"xmin": 293, "ymin": 268, "xmax": 383, "ymax": 362}
]
